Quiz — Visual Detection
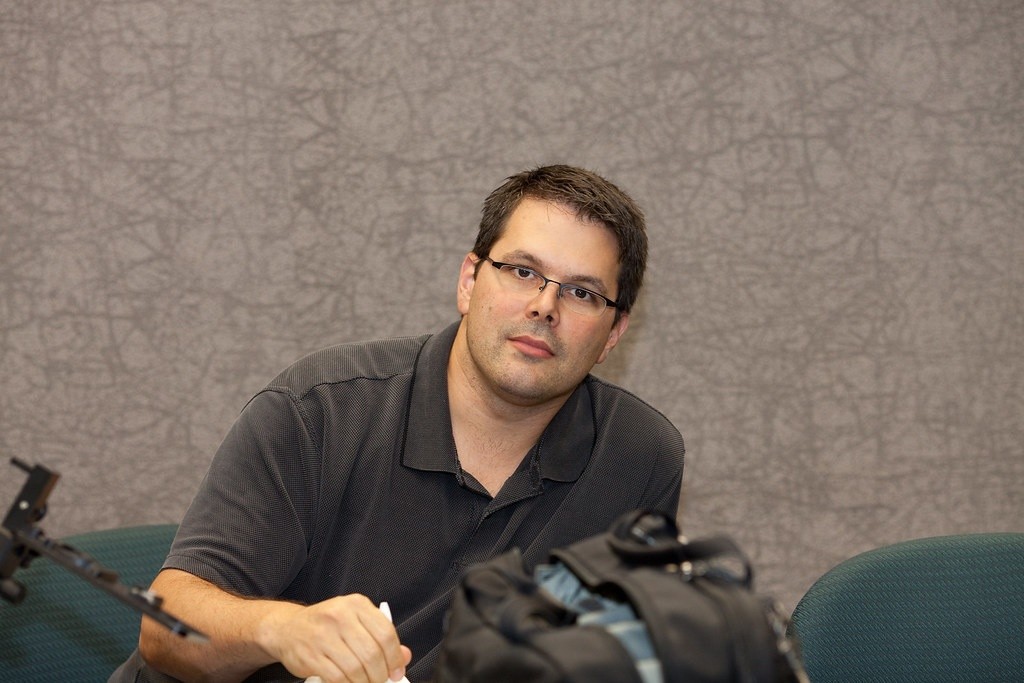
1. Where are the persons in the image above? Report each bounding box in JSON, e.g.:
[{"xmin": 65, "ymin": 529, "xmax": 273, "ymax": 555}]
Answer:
[{"xmin": 106, "ymin": 165, "xmax": 684, "ymax": 683}]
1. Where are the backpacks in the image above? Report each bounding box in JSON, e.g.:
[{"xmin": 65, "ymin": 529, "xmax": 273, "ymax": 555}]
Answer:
[{"xmin": 410, "ymin": 508, "xmax": 797, "ymax": 683}]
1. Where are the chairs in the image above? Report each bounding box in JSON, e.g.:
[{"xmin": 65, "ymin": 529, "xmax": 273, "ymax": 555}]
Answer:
[
  {"xmin": 786, "ymin": 533, "xmax": 1024, "ymax": 683},
  {"xmin": 0, "ymin": 522, "xmax": 179, "ymax": 683}
]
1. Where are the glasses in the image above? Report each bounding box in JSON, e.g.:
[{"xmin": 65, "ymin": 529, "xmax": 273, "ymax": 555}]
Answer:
[{"xmin": 485, "ymin": 256, "xmax": 616, "ymax": 317}]
